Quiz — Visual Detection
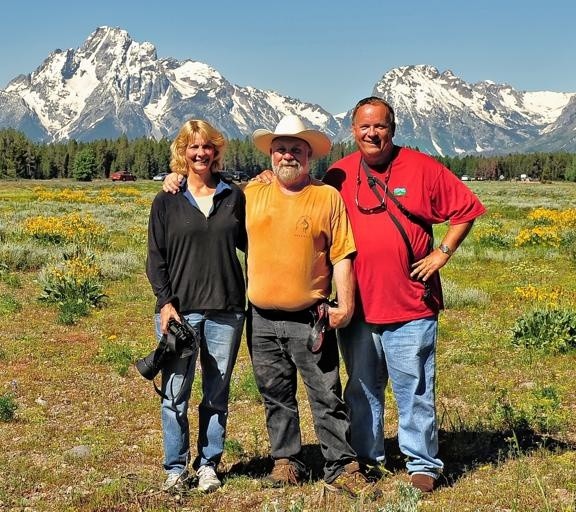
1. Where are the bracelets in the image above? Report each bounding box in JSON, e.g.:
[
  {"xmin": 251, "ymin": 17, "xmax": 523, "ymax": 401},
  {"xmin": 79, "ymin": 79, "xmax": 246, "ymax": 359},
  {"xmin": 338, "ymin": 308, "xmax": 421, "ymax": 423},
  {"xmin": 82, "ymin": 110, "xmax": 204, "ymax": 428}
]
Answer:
[{"xmin": 438, "ymin": 243, "xmax": 454, "ymax": 257}]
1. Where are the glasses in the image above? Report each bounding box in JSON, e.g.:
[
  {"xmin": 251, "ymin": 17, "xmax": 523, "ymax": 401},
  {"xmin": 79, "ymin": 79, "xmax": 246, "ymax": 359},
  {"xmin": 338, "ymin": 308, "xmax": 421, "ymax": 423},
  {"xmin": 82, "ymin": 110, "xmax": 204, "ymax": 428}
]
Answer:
[{"xmin": 356, "ymin": 184, "xmax": 389, "ymax": 213}]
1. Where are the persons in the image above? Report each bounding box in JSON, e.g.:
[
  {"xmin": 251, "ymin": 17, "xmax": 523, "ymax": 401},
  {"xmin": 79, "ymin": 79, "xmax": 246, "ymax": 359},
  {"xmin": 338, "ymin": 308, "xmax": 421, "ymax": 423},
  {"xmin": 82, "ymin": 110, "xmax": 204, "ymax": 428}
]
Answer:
[
  {"xmin": 160, "ymin": 114, "xmax": 384, "ymax": 500},
  {"xmin": 143, "ymin": 118, "xmax": 248, "ymax": 495},
  {"xmin": 250, "ymin": 96, "xmax": 488, "ymax": 494}
]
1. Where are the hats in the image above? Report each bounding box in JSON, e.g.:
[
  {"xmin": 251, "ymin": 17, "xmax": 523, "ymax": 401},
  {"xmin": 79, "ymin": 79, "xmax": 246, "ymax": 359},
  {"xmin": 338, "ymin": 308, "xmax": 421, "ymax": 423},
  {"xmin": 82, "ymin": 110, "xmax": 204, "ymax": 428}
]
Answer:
[{"xmin": 252, "ymin": 114, "xmax": 331, "ymax": 160}]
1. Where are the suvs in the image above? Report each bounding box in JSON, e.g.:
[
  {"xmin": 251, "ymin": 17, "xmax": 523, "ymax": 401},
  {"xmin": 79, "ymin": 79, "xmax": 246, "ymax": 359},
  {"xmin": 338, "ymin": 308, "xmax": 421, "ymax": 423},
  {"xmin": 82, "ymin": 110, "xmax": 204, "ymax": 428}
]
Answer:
[{"xmin": 231, "ymin": 171, "xmax": 251, "ymax": 183}]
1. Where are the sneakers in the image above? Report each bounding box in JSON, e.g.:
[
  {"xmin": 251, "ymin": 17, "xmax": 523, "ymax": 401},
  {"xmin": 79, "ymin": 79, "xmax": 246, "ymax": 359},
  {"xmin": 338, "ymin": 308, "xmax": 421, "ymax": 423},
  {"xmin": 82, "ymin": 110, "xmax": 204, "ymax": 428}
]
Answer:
[
  {"xmin": 410, "ymin": 472, "xmax": 437, "ymax": 491},
  {"xmin": 263, "ymin": 458, "xmax": 302, "ymax": 486},
  {"xmin": 324, "ymin": 462, "xmax": 383, "ymax": 500},
  {"xmin": 196, "ymin": 465, "xmax": 222, "ymax": 488},
  {"xmin": 164, "ymin": 472, "xmax": 186, "ymax": 492}
]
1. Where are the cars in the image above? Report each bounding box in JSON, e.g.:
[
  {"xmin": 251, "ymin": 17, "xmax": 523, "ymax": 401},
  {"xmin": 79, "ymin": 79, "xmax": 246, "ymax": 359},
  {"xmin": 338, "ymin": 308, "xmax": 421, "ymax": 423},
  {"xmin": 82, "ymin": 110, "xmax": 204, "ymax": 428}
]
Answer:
[
  {"xmin": 459, "ymin": 173, "xmax": 533, "ymax": 183},
  {"xmin": 110, "ymin": 171, "xmax": 138, "ymax": 181},
  {"xmin": 216, "ymin": 169, "xmax": 232, "ymax": 183},
  {"xmin": 152, "ymin": 171, "xmax": 170, "ymax": 181}
]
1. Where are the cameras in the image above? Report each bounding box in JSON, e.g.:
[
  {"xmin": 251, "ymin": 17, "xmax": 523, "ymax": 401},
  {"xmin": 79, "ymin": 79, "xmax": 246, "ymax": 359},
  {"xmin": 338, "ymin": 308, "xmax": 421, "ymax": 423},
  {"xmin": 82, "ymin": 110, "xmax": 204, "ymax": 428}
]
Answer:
[
  {"xmin": 308, "ymin": 291, "xmax": 337, "ymax": 328},
  {"xmin": 136, "ymin": 314, "xmax": 198, "ymax": 381}
]
